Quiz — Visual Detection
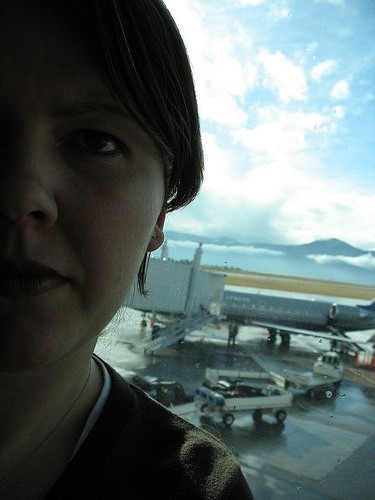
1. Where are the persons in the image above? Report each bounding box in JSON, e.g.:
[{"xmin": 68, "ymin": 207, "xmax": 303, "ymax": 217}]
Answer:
[
  {"xmin": 227, "ymin": 323, "xmax": 240, "ymax": 348},
  {"xmin": 0, "ymin": 2, "xmax": 253, "ymax": 500}
]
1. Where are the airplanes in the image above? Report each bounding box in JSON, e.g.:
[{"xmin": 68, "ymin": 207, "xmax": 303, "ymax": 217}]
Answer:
[{"xmin": 138, "ymin": 283, "xmax": 375, "ymax": 369}]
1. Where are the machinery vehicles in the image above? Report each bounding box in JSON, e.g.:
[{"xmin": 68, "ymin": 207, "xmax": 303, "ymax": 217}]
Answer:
[{"xmin": 283, "ymin": 349, "xmax": 344, "ymax": 401}]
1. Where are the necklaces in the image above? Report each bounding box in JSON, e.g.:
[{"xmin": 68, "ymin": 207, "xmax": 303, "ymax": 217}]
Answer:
[{"xmin": 0, "ymin": 357, "xmax": 92, "ymax": 485}]
